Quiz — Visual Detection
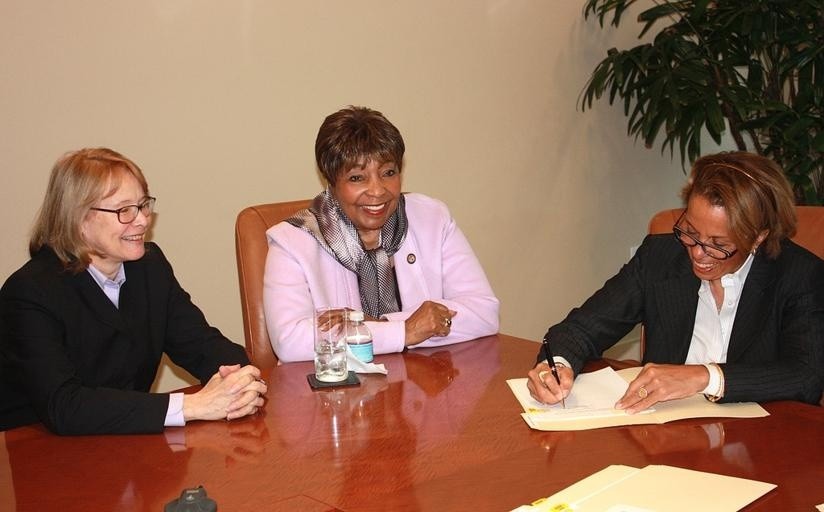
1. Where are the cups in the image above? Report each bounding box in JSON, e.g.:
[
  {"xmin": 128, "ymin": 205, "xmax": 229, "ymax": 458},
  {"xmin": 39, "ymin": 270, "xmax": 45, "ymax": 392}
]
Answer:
[{"xmin": 314, "ymin": 307, "xmax": 347, "ymax": 382}]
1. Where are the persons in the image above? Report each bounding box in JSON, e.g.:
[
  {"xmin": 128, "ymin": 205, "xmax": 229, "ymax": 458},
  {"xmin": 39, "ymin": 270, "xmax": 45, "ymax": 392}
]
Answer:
[
  {"xmin": 262, "ymin": 105, "xmax": 500, "ymax": 362},
  {"xmin": 527, "ymin": 151, "xmax": 824, "ymax": 414},
  {"xmin": 0, "ymin": 148, "xmax": 267, "ymax": 435}
]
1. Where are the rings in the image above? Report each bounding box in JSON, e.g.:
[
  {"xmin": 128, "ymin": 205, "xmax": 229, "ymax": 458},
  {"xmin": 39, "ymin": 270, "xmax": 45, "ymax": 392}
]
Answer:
[
  {"xmin": 444, "ymin": 318, "xmax": 451, "ymax": 327},
  {"xmin": 638, "ymin": 388, "xmax": 647, "ymax": 398},
  {"xmin": 538, "ymin": 371, "xmax": 548, "ymax": 381}
]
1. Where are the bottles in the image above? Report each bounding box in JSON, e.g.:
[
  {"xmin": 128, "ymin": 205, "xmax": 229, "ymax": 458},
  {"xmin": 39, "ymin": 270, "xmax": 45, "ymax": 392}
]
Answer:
[{"xmin": 347, "ymin": 311, "xmax": 374, "ymax": 362}]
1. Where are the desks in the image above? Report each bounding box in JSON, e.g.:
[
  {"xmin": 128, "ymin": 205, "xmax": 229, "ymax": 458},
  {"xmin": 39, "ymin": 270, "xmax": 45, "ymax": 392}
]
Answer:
[{"xmin": 0, "ymin": 331, "xmax": 823, "ymax": 512}]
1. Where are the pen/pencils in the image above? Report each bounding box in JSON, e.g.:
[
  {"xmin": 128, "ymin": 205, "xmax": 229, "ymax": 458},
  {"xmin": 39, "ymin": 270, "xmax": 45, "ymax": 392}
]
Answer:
[{"xmin": 543, "ymin": 338, "xmax": 566, "ymax": 408}]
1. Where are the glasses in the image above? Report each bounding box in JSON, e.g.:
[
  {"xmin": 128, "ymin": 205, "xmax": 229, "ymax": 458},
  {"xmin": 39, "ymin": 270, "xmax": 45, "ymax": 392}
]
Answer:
[
  {"xmin": 87, "ymin": 196, "xmax": 156, "ymax": 223},
  {"xmin": 674, "ymin": 209, "xmax": 738, "ymax": 260}
]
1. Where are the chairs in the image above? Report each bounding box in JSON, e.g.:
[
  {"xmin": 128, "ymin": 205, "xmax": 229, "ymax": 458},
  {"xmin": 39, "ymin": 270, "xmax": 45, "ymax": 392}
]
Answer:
[
  {"xmin": 634, "ymin": 206, "xmax": 824, "ymax": 366},
  {"xmin": 235, "ymin": 193, "xmax": 416, "ymax": 367}
]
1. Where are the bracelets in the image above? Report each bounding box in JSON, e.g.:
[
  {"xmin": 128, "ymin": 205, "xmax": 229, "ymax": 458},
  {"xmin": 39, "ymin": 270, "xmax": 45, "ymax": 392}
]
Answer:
[
  {"xmin": 554, "ymin": 363, "xmax": 566, "ymax": 369},
  {"xmin": 707, "ymin": 363, "xmax": 724, "ymax": 402}
]
